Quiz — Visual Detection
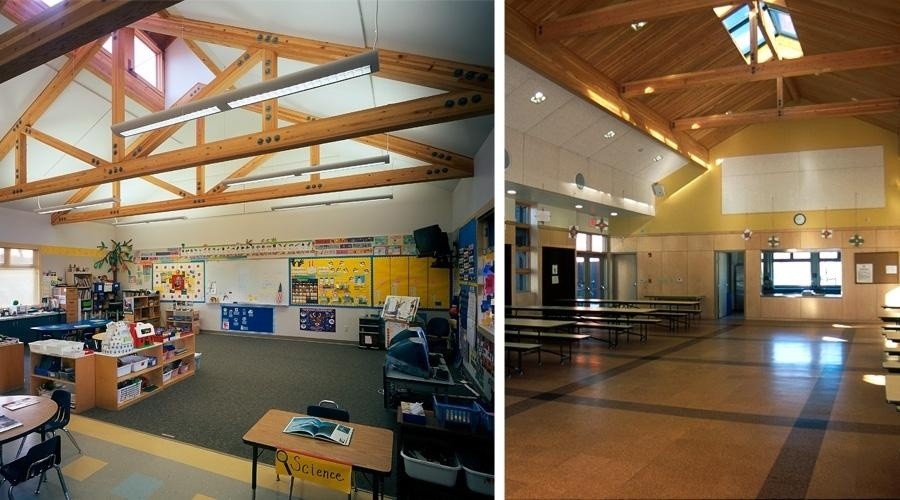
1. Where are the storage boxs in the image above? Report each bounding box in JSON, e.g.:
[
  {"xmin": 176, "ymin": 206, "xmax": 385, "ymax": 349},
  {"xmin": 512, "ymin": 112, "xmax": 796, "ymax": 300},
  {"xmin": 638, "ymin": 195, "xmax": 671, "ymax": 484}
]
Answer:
[
  {"xmin": 462, "ymin": 465, "xmax": 494, "ymax": 498},
  {"xmin": 400, "ymin": 439, "xmax": 461, "ymax": 486}
]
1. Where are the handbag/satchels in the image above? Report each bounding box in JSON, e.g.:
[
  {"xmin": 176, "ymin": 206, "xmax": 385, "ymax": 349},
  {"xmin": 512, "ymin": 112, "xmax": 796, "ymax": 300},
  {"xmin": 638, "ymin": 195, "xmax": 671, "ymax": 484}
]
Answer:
[
  {"xmin": 130, "ymin": 322, "xmax": 156, "ymax": 348},
  {"xmin": 101, "ymin": 320, "xmax": 134, "ymax": 355}
]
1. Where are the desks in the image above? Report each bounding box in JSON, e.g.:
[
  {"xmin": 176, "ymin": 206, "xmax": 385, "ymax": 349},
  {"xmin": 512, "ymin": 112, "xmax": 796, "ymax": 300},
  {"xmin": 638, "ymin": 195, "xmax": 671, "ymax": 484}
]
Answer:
[
  {"xmin": 243, "ymin": 409, "xmax": 394, "ymax": 500},
  {"xmin": 0, "ymin": 395, "xmax": 59, "ymax": 482},
  {"xmin": 383, "ymin": 353, "xmax": 455, "ymax": 408}
]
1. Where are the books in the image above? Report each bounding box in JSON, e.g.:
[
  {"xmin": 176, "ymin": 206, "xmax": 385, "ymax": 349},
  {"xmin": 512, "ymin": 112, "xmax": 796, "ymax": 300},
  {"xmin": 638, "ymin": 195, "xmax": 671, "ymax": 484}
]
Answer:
[
  {"xmin": 283, "ymin": 417, "xmax": 354, "ymax": 445},
  {"xmin": 0, "ymin": 415, "xmax": 22, "ymax": 433},
  {"xmin": 75, "ymin": 276, "xmax": 91, "ymax": 299},
  {"xmin": 36, "ymin": 384, "xmax": 76, "ymax": 408},
  {"xmin": 1, "ymin": 397, "xmax": 40, "ymax": 411}
]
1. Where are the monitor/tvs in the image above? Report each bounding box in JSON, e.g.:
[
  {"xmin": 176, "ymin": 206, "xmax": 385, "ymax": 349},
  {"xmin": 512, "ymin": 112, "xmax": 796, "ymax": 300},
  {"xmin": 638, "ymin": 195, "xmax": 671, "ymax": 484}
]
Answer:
[{"xmin": 414, "ymin": 224, "xmax": 441, "ymax": 253}]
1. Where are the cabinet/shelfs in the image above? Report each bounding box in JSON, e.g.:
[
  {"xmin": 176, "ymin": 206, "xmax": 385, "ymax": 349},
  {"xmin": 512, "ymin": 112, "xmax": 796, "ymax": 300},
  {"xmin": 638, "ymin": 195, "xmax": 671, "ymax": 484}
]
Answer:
[
  {"xmin": 0, "ymin": 270, "xmax": 200, "ymax": 415},
  {"xmin": 358, "ymin": 317, "xmax": 382, "ymax": 350},
  {"xmin": 396, "ymin": 406, "xmax": 494, "ymax": 500}
]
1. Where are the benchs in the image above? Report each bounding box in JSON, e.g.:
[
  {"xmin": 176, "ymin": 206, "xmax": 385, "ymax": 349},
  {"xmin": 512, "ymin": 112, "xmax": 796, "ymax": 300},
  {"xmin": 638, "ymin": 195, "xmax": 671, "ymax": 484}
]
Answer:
[
  {"xmin": 505, "ymin": 294, "xmax": 703, "ymax": 376},
  {"xmin": 877, "ymin": 301, "xmax": 900, "ymax": 412}
]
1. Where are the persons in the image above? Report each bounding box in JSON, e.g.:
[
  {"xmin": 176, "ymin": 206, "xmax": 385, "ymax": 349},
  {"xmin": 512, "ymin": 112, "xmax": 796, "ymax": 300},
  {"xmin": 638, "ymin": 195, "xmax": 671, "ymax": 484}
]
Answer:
[{"xmin": 762, "ymin": 276, "xmax": 773, "ymax": 295}]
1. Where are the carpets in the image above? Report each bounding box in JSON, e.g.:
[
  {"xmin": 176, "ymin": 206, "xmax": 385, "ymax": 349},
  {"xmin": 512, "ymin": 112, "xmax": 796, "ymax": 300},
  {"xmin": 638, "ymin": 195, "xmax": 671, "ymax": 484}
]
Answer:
[{"xmin": 80, "ymin": 332, "xmax": 396, "ymax": 497}]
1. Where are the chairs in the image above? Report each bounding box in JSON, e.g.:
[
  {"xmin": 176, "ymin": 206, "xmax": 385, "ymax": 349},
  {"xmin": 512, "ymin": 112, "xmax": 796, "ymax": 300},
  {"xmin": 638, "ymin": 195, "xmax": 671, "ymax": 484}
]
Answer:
[
  {"xmin": 425, "ymin": 318, "xmax": 450, "ymax": 359},
  {"xmin": 0, "ymin": 435, "xmax": 70, "ymax": 500},
  {"xmin": 288, "ymin": 401, "xmax": 357, "ymax": 500},
  {"xmin": 15, "ymin": 389, "xmax": 81, "ymax": 482}
]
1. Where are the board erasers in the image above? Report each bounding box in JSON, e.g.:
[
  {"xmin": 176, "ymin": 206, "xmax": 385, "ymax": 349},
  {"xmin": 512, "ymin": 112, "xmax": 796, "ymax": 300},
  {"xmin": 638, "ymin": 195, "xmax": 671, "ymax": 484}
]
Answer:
[{"xmin": 233, "ymin": 302, "xmax": 237, "ymax": 304}]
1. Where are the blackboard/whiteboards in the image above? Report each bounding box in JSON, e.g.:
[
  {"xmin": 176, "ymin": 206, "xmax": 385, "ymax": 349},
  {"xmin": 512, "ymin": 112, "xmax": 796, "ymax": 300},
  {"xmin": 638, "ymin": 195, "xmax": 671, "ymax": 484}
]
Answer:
[
  {"xmin": 153, "ymin": 262, "xmax": 204, "ymax": 303},
  {"xmin": 205, "ymin": 259, "xmax": 289, "ymax": 307}
]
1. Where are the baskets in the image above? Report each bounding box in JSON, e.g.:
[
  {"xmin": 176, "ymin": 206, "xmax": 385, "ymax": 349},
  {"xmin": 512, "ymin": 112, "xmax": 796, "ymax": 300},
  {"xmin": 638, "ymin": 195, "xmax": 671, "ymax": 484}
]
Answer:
[{"xmin": 117, "ymin": 380, "xmax": 142, "ymax": 404}]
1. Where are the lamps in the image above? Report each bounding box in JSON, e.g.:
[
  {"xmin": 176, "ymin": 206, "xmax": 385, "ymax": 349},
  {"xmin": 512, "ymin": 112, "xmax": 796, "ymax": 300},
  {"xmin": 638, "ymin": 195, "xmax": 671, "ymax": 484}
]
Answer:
[{"xmin": 34, "ymin": 1, "xmax": 394, "ymax": 226}]
